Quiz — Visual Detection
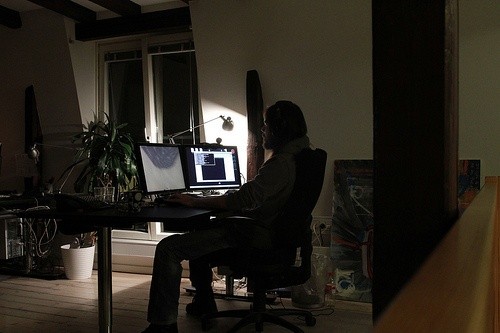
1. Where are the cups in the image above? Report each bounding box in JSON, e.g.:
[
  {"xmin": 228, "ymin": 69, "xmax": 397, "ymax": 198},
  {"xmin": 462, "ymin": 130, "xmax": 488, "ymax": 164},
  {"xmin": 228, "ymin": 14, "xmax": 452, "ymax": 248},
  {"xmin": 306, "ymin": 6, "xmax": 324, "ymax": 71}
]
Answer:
[{"xmin": 94, "ymin": 186, "xmax": 115, "ymax": 202}]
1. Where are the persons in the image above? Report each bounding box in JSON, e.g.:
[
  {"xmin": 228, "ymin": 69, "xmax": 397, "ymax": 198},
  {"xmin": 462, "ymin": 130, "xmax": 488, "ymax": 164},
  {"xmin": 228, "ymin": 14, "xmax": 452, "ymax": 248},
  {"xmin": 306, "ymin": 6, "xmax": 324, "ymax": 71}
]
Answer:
[{"xmin": 140, "ymin": 100, "xmax": 313, "ymax": 333}]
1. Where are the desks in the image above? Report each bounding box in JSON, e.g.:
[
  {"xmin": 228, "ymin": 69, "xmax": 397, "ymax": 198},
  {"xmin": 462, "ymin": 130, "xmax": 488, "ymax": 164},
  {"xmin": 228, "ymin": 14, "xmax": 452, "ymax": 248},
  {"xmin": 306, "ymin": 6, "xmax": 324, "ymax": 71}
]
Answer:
[{"xmin": 75, "ymin": 207, "xmax": 216, "ymax": 333}]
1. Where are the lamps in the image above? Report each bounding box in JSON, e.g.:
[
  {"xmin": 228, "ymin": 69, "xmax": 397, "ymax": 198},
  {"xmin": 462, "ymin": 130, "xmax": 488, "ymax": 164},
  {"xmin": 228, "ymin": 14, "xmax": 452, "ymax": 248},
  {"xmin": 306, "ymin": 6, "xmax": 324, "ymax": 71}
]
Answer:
[{"xmin": 169, "ymin": 116, "xmax": 233, "ymax": 144}]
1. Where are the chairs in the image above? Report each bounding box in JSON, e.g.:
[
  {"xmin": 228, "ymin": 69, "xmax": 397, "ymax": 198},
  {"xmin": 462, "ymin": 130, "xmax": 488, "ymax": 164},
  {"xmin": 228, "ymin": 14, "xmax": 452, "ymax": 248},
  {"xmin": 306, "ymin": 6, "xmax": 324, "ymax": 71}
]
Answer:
[{"xmin": 192, "ymin": 147, "xmax": 327, "ymax": 333}]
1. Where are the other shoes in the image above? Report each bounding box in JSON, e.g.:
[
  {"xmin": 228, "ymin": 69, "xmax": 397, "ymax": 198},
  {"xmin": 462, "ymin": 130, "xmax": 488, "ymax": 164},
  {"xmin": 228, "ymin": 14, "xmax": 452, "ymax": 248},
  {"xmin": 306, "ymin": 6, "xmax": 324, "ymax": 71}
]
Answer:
[
  {"xmin": 141, "ymin": 322, "xmax": 179, "ymax": 333},
  {"xmin": 186, "ymin": 298, "xmax": 219, "ymax": 321}
]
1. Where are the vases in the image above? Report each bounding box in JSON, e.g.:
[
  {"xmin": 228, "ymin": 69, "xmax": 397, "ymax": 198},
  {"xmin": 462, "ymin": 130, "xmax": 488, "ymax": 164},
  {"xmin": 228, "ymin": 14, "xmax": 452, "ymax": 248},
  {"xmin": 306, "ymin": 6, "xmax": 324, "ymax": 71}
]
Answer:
[{"xmin": 60, "ymin": 243, "xmax": 95, "ymax": 279}]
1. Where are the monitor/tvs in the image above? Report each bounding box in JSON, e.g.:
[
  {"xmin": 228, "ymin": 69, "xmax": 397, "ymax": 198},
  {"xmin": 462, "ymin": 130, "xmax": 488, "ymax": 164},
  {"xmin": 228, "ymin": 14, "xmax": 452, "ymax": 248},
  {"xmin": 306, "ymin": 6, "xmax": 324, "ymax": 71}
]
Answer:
[{"xmin": 136, "ymin": 143, "xmax": 242, "ymax": 194}]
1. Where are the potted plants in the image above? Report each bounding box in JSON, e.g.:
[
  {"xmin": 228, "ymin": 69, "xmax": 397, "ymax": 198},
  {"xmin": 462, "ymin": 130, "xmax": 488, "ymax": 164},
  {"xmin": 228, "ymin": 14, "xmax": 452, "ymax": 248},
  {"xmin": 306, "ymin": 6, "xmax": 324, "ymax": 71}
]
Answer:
[{"xmin": 60, "ymin": 110, "xmax": 142, "ymax": 202}]
